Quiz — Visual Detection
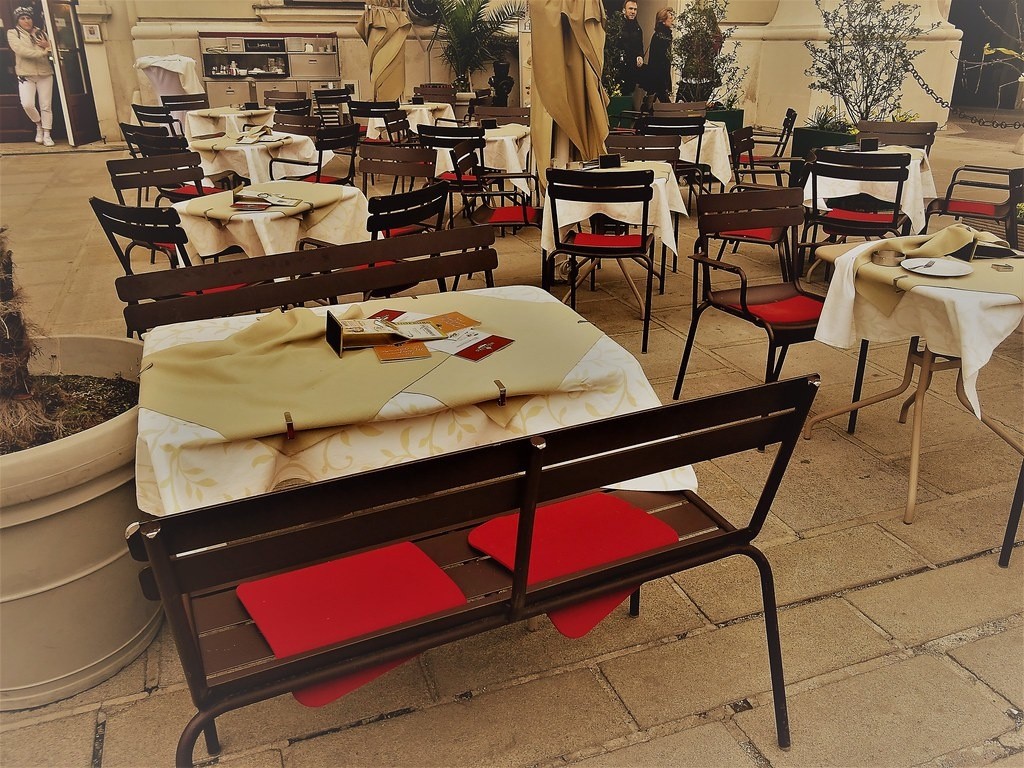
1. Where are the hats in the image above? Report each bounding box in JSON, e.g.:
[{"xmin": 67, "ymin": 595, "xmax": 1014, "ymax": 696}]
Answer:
[{"xmin": 13, "ymin": 7, "xmax": 32, "ymax": 23}]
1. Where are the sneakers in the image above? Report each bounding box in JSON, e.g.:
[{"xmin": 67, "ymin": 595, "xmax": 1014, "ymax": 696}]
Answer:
[
  {"xmin": 42, "ymin": 136, "xmax": 54, "ymax": 147},
  {"xmin": 35, "ymin": 129, "xmax": 43, "ymax": 143}
]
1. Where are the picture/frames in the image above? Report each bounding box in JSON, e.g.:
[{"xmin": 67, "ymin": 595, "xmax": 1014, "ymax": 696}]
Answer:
[{"xmin": 80, "ymin": 20, "xmax": 103, "ymax": 44}]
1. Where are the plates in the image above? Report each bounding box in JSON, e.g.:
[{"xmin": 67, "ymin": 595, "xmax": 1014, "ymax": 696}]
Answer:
[{"xmin": 900, "ymin": 257, "xmax": 973, "ymax": 276}]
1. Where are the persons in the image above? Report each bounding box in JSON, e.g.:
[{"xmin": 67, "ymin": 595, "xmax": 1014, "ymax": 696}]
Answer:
[
  {"xmin": 616, "ymin": 0, "xmax": 644, "ymax": 96},
  {"xmin": 8, "ymin": 6, "xmax": 54, "ymax": 146},
  {"xmin": 641, "ymin": 8, "xmax": 676, "ymax": 111},
  {"xmin": 676, "ymin": 9, "xmax": 723, "ymax": 103}
]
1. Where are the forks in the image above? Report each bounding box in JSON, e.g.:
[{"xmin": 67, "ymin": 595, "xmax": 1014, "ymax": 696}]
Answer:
[{"xmin": 906, "ymin": 260, "xmax": 936, "ymax": 271}]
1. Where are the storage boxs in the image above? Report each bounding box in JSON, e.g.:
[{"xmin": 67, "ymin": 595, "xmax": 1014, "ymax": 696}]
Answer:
[
  {"xmin": 225, "ymin": 36, "xmax": 244, "ymax": 51},
  {"xmin": 287, "ymin": 36, "xmax": 305, "ymax": 51},
  {"xmin": 305, "ymin": 44, "xmax": 313, "ymax": 52}
]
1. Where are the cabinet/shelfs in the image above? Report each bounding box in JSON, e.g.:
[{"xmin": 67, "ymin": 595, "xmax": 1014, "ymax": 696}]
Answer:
[
  {"xmin": 255, "ymin": 81, "xmax": 312, "ymax": 110},
  {"xmin": 196, "ymin": 34, "xmax": 341, "ymax": 81},
  {"xmin": 202, "ymin": 82, "xmax": 254, "ymax": 106}
]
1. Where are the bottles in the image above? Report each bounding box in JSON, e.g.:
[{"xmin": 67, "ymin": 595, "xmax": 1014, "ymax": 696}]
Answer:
[{"xmin": 211, "ymin": 64, "xmax": 228, "ymax": 75}]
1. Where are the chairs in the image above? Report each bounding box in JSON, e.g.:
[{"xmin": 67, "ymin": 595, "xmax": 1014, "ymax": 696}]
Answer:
[
  {"xmin": 806, "ymin": 144, "xmax": 912, "ymax": 266},
  {"xmin": 85, "ymin": 83, "xmax": 1024, "ymax": 567}
]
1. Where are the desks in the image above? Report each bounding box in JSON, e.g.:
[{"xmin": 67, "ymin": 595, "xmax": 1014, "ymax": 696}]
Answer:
[
  {"xmin": 432, "ymin": 124, "xmax": 531, "ymax": 237},
  {"xmin": 136, "ymin": 54, "xmax": 204, "ymax": 135},
  {"xmin": 798, "ymin": 140, "xmax": 933, "ymax": 284},
  {"xmin": 364, "ymin": 102, "xmax": 456, "ymax": 139},
  {"xmin": 171, "ymin": 177, "xmax": 366, "ymax": 313},
  {"xmin": 804, "ymin": 223, "xmax": 1024, "ymax": 530},
  {"xmin": 184, "ymin": 103, "xmax": 274, "ymax": 132},
  {"xmin": 536, "ymin": 154, "xmax": 680, "ymax": 322},
  {"xmin": 126, "ymin": 282, "xmax": 704, "ymax": 558},
  {"xmin": 644, "ymin": 119, "xmax": 733, "ymax": 193},
  {"xmin": 187, "ymin": 128, "xmax": 336, "ymax": 188}
]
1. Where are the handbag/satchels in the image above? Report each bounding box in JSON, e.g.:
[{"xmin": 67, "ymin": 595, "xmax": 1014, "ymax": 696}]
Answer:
[{"xmin": 635, "ymin": 63, "xmax": 650, "ymax": 87}]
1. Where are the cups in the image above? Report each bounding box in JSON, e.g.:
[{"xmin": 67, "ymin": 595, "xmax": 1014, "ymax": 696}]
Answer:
[{"xmin": 229, "ymin": 68, "xmax": 240, "ymax": 75}]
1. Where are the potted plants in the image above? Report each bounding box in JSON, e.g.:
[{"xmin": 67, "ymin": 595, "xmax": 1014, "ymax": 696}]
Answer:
[
  {"xmin": 656, "ymin": 0, "xmax": 750, "ymax": 183},
  {"xmin": 0, "ymin": 224, "xmax": 167, "ymax": 713},
  {"xmin": 602, "ymin": 6, "xmax": 641, "ymax": 126},
  {"xmin": 786, "ymin": 0, "xmax": 949, "ymax": 187}
]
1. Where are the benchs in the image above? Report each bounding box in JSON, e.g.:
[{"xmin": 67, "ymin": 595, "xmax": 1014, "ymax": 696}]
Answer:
[
  {"xmin": 118, "ymin": 370, "xmax": 824, "ymax": 768},
  {"xmin": 112, "ymin": 224, "xmax": 501, "ymax": 341}
]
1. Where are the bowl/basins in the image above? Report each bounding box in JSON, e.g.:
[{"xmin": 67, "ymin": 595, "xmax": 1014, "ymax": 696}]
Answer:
[{"xmin": 872, "ymin": 249, "xmax": 906, "ymax": 267}]
